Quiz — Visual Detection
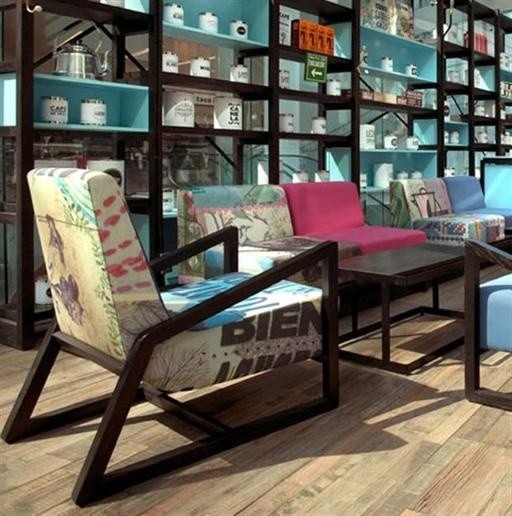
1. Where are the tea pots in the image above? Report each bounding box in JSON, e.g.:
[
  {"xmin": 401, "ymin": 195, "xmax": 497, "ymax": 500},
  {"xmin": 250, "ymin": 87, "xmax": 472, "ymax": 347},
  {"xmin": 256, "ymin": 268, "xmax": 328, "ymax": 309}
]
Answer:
[{"xmin": 53, "ymin": 19, "xmax": 112, "ymax": 80}]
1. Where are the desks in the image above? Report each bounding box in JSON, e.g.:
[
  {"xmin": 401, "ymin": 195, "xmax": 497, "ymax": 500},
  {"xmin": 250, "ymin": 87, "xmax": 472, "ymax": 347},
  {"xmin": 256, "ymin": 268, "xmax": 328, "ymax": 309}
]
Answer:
[{"xmin": 337, "ymin": 243, "xmax": 465, "ymax": 374}]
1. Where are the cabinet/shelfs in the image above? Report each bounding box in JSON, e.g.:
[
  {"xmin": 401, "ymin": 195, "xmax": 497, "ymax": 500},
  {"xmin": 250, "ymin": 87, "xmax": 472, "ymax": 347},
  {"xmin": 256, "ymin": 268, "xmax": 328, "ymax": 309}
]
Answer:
[{"xmin": 0, "ymin": 0, "xmax": 512, "ymax": 352}]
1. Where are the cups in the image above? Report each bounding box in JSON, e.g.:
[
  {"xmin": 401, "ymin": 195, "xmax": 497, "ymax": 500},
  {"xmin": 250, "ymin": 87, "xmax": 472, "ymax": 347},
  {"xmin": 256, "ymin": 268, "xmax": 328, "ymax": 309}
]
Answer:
[
  {"xmin": 293, "ymin": 170, "xmax": 309, "ymax": 184},
  {"xmin": 326, "ymin": 80, "xmax": 342, "ymax": 96},
  {"xmin": 39, "ymin": 94, "xmax": 109, "ymax": 126},
  {"xmin": 310, "ymin": 116, "xmax": 328, "ymax": 135},
  {"xmin": 314, "ymin": 170, "xmax": 330, "ymax": 182},
  {"xmin": 212, "ymin": 95, "xmax": 244, "ymax": 130},
  {"xmin": 406, "ymin": 137, "xmax": 420, "ymax": 150},
  {"xmin": 162, "ymin": 3, "xmax": 186, "ymax": 26},
  {"xmin": 227, "ymin": 64, "xmax": 251, "ymax": 84},
  {"xmin": 360, "ymin": 44, "xmax": 370, "ymax": 66},
  {"xmin": 230, "ymin": 18, "xmax": 248, "ymax": 39},
  {"xmin": 166, "ymin": 90, "xmax": 196, "ymax": 129},
  {"xmin": 397, "ymin": 171, "xmax": 409, "ymax": 179},
  {"xmin": 411, "ymin": 171, "xmax": 422, "ymax": 179},
  {"xmin": 279, "ymin": 112, "xmax": 296, "ymax": 133},
  {"xmin": 190, "ymin": 57, "xmax": 213, "ymax": 78},
  {"xmin": 361, "ymin": 170, "xmax": 368, "ymax": 188},
  {"xmin": 404, "ymin": 63, "xmax": 418, "ymax": 76},
  {"xmin": 278, "ymin": 69, "xmax": 291, "ymax": 89},
  {"xmin": 380, "ymin": 56, "xmax": 394, "ymax": 72},
  {"xmin": 373, "ymin": 162, "xmax": 394, "ymax": 187},
  {"xmin": 196, "ymin": 11, "xmax": 219, "ymax": 34},
  {"xmin": 440, "ymin": 4, "xmax": 488, "ymax": 179},
  {"xmin": 383, "ymin": 135, "xmax": 398, "ymax": 150},
  {"xmin": 163, "ymin": 50, "xmax": 181, "ymax": 74}
]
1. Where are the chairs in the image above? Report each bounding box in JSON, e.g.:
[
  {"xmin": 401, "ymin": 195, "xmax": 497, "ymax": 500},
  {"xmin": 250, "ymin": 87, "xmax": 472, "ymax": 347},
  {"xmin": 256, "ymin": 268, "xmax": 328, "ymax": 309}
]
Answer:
[
  {"xmin": 0, "ymin": 168, "xmax": 339, "ymax": 508},
  {"xmin": 389, "ymin": 178, "xmax": 506, "ymax": 246},
  {"xmin": 461, "ymin": 239, "xmax": 512, "ymax": 410},
  {"xmin": 443, "ymin": 176, "xmax": 512, "ymax": 230},
  {"xmin": 280, "ymin": 181, "xmax": 426, "ymax": 255},
  {"xmin": 175, "ymin": 186, "xmax": 359, "ymax": 289}
]
